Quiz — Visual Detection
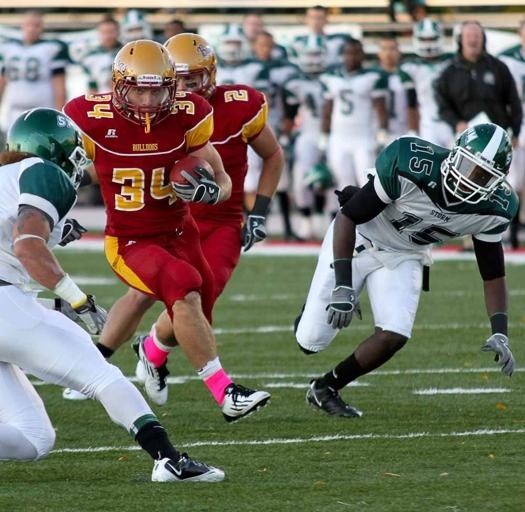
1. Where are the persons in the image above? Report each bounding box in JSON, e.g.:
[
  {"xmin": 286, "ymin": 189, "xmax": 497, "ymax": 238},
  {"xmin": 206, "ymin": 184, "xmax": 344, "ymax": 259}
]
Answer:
[
  {"xmin": 293, "ymin": 123, "xmax": 520, "ymax": 419},
  {"xmin": 1, "ymin": 108, "xmax": 224, "ymax": 484},
  {"xmin": 1, "ymin": 0, "xmax": 421, "ymax": 251},
  {"xmin": 405, "ymin": 1, "xmax": 524, "ymax": 251}
]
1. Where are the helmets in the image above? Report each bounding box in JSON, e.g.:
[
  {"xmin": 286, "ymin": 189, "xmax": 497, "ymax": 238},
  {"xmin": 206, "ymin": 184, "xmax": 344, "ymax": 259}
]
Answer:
[
  {"xmin": 162, "ymin": 40, "xmax": 218, "ymax": 97},
  {"xmin": 439, "ymin": 122, "xmax": 513, "ymax": 207},
  {"xmin": 109, "ymin": 40, "xmax": 178, "ymax": 114},
  {"xmin": 4, "ymin": 105, "xmax": 88, "ymax": 192}
]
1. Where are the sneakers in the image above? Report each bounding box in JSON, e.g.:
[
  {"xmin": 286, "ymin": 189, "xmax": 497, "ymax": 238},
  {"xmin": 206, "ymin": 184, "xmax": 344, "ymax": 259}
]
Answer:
[
  {"xmin": 219, "ymin": 382, "xmax": 272, "ymax": 425},
  {"xmin": 130, "ymin": 334, "xmax": 169, "ymax": 407},
  {"xmin": 151, "ymin": 451, "xmax": 226, "ymax": 484},
  {"xmin": 304, "ymin": 377, "xmax": 364, "ymax": 419}
]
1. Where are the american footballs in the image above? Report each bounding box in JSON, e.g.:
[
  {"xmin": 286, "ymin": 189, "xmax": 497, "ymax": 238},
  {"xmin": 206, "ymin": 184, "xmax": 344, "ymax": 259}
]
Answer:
[{"xmin": 169, "ymin": 156, "xmax": 215, "ymax": 184}]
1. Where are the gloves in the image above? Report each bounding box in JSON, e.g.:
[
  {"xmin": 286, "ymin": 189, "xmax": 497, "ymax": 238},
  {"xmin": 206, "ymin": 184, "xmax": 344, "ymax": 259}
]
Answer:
[
  {"xmin": 52, "ymin": 273, "xmax": 109, "ymax": 337},
  {"xmin": 171, "ymin": 166, "xmax": 221, "ymax": 207},
  {"xmin": 241, "ymin": 194, "xmax": 272, "ymax": 253},
  {"xmin": 480, "ymin": 311, "xmax": 516, "ymax": 378},
  {"xmin": 325, "ymin": 257, "xmax": 363, "ymax": 330},
  {"xmin": 58, "ymin": 215, "xmax": 88, "ymax": 247}
]
